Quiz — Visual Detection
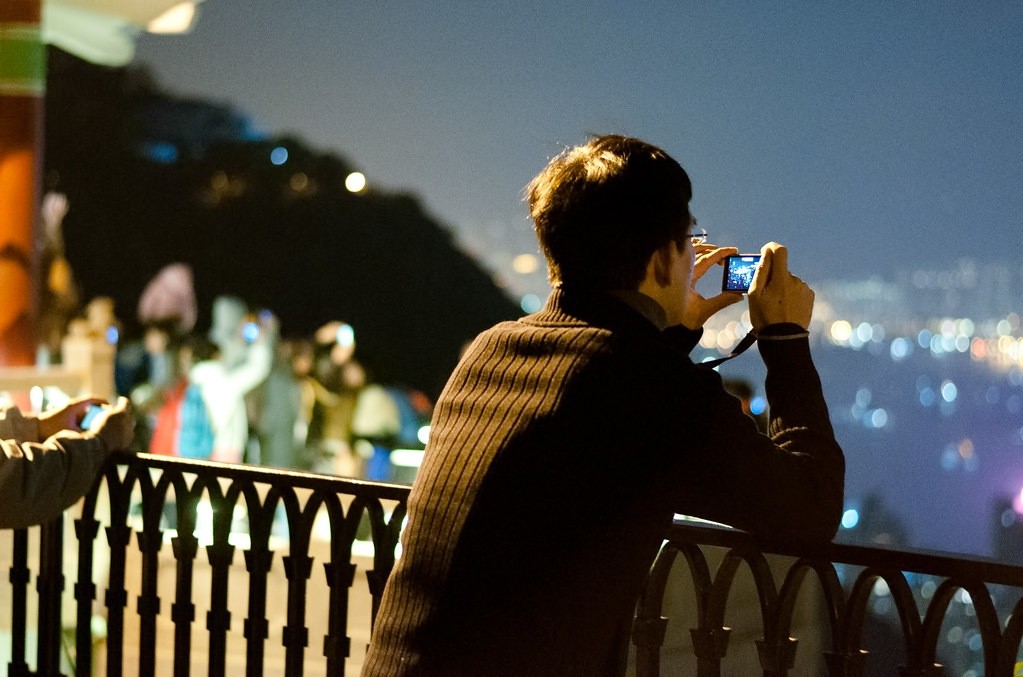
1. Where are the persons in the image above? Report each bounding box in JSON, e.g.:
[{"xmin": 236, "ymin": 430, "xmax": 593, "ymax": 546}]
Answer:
[
  {"xmin": 360, "ymin": 135, "xmax": 846, "ymax": 677},
  {"xmin": 32, "ymin": 195, "xmax": 422, "ymax": 479},
  {"xmin": 0, "ymin": 394, "xmax": 136, "ymax": 529}
]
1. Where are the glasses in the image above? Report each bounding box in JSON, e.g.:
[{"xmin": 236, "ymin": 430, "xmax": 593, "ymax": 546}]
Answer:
[{"xmin": 686, "ymin": 225, "xmax": 708, "ymax": 248}]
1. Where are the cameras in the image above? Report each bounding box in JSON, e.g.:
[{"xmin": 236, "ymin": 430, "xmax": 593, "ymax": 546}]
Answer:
[
  {"xmin": 721, "ymin": 254, "xmax": 763, "ymax": 295},
  {"xmin": 80, "ymin": 406, "xmax": 104, "ymax": 430}
]
